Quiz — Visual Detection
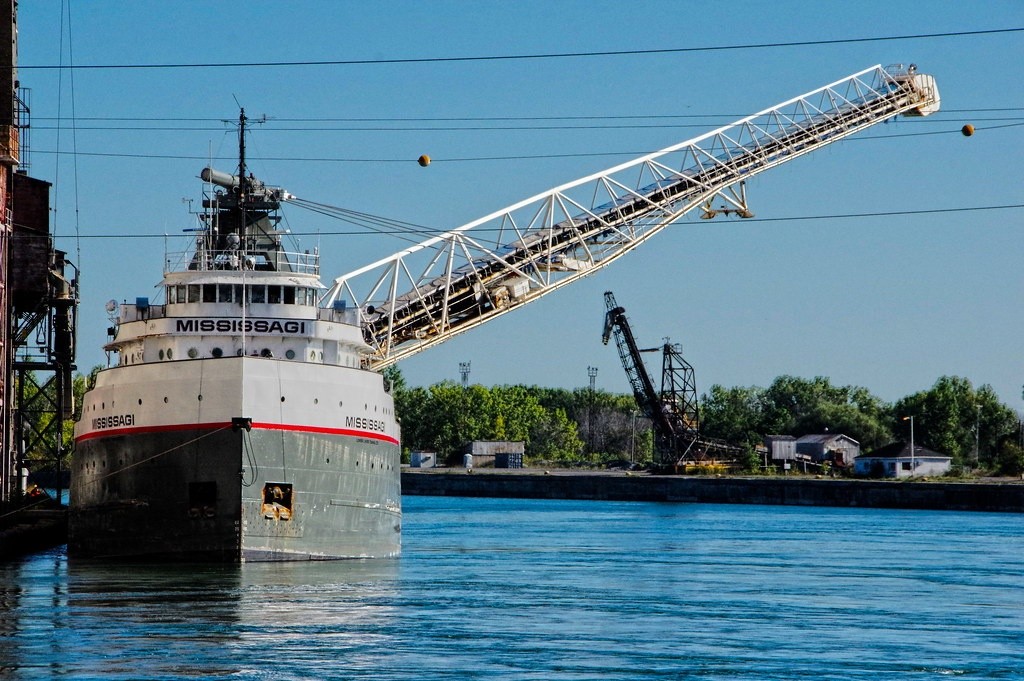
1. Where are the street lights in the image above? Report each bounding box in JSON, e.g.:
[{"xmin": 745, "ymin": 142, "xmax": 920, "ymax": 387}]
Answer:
[{"xmin": 903, "ymin": 416, "xmax": 914, "ymax": 477}]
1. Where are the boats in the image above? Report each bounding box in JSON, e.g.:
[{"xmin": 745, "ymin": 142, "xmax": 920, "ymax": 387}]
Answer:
[{"xmin": 67, "ymin": 57, "xmax": 941, "ymax": 563}]
